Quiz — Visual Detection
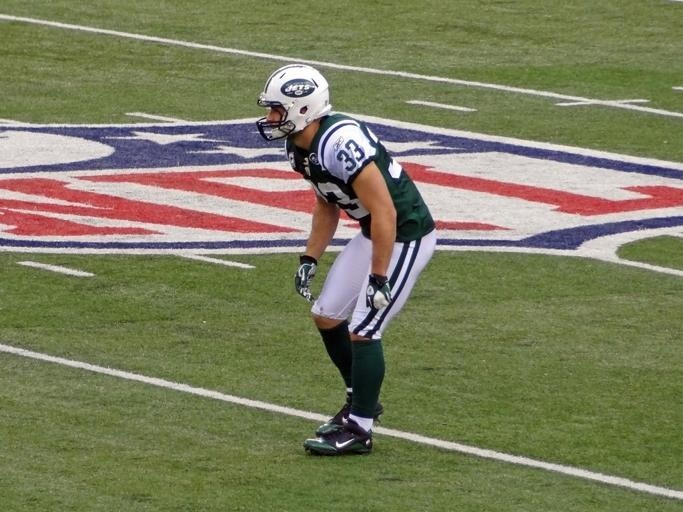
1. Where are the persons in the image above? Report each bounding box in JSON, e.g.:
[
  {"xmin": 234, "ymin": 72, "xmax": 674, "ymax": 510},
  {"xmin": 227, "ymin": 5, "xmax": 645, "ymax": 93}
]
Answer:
[{"xmin": 256, "ymin": 64, "xmax": 437, "ymax": 455}]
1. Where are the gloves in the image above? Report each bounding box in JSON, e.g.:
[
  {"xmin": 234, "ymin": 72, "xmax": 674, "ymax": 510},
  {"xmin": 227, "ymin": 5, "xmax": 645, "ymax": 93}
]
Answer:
[
  {"xmin": 294, "ymin": 255, "xmax": 318, "ymax": 303},
  {"xmin": 365, "ymin": 273, "xmax": 393, "ymax": 311}
]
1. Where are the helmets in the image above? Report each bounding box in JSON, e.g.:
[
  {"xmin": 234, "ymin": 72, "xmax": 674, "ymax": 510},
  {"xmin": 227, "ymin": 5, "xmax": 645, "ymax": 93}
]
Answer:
[{"xmin": 255, "ymin": 64, "xmax": 335, "ymax": 141}]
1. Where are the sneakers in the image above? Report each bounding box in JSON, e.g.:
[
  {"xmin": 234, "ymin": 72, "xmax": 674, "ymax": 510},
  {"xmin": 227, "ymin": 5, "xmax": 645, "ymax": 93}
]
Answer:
[
  {"xmin": 316, "ymin": 395, "xmax": 384, "ymax": 437},
  {"xmin": 304, "ymin": 420, "xmax": 373, "ymax": 456}
]
